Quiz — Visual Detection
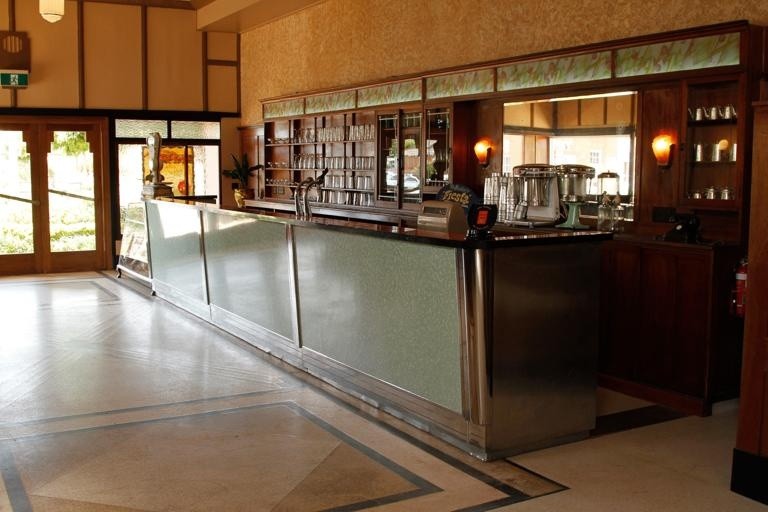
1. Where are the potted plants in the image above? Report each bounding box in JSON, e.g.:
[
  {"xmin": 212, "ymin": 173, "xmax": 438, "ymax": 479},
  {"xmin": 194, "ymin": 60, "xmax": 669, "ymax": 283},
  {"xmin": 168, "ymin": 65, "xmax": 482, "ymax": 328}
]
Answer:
[{"xmin": 223, "ymin": 153, "xmax": 264, "ymax": 200}]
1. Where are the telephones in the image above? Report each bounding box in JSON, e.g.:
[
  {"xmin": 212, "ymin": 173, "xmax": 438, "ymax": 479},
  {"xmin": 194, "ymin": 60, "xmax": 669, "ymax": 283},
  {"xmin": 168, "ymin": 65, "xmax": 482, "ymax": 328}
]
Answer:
[{"xmin": 667, "ymin": 212, "xmax": 700, "ymax": 243}]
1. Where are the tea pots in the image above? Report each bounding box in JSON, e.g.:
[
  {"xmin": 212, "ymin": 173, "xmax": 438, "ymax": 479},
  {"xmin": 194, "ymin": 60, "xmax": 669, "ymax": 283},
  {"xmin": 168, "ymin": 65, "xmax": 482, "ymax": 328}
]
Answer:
[{"xmin": 686, "ymin": 103, "xmax": 739, "ymax": 200}]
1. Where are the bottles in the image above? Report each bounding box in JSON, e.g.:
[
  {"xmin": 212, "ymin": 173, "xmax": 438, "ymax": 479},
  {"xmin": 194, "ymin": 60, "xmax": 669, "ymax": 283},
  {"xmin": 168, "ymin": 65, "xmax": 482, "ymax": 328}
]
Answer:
[
  {"xmin": 597, "ymin": 194, "xmax": 613, "ymax": 232},
  {"xmin": 597, "ymin": 170, "xmax": 621, "ymax": 204},
  {"xmin": 610, "ymin": 192, "xmax": 625, "ymax": 233}
]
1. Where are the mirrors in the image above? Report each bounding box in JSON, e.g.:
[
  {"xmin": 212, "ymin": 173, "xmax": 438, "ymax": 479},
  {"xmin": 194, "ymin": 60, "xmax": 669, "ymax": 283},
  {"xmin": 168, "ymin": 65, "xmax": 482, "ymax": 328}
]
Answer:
[{"xmin": 501, "ymin": 91, "xmax": 638, "ymax": 222}]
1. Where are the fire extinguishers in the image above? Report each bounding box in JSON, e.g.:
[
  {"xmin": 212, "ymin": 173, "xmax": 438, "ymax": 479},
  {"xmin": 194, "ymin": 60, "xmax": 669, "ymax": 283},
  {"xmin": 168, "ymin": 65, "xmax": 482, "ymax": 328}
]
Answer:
[{"xmin": 731, "ymin": 257, "xmax": 747, "ymax": 316}]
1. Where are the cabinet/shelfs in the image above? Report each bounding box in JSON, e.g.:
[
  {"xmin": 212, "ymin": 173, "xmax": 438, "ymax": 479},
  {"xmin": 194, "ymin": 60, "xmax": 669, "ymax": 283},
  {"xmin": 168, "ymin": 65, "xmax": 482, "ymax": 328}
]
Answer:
[
  {"xmin": 264, "ymin": 101, "xmax": 469, "ymax": 210},
  {"xmin": 677, "ymin": 72, "xmax": 753, "ymax": 211}
]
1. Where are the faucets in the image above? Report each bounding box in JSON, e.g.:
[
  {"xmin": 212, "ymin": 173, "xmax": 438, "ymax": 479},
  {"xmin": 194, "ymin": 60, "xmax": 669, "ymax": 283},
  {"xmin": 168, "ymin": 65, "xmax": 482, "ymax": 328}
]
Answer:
[
  {"xmin": 303, "ymin": 180, "xmax": 324, "ymax": 218},
  {"xmin": 293, "ymin": 176, "xmax": 313, "ymax": 217}
]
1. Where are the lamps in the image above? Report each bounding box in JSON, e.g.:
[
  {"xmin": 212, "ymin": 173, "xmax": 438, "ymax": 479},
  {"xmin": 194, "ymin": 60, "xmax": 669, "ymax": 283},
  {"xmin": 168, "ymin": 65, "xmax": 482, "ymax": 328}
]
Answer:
[
  {"xmin": 474, "ymin": 139, "xmax": 491, "ymax": 168},
  {"xmin": 39, "ymin": 0, "xmax": 65, "ymax": 23},
  {"xmin": 652, "ymin": 135, "xmax": 674, "ymax": 169}
]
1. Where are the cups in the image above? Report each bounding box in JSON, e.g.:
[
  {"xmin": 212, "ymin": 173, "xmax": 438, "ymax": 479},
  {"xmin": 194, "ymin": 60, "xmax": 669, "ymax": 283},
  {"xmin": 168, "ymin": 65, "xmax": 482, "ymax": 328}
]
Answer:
[
  {"xmin": 264, "ymin": 123, "xmax": 376, "ymax": 207},
  {"xmin": 482, "ymin": 171, "xmax": 528, "ymax": 225}
]
